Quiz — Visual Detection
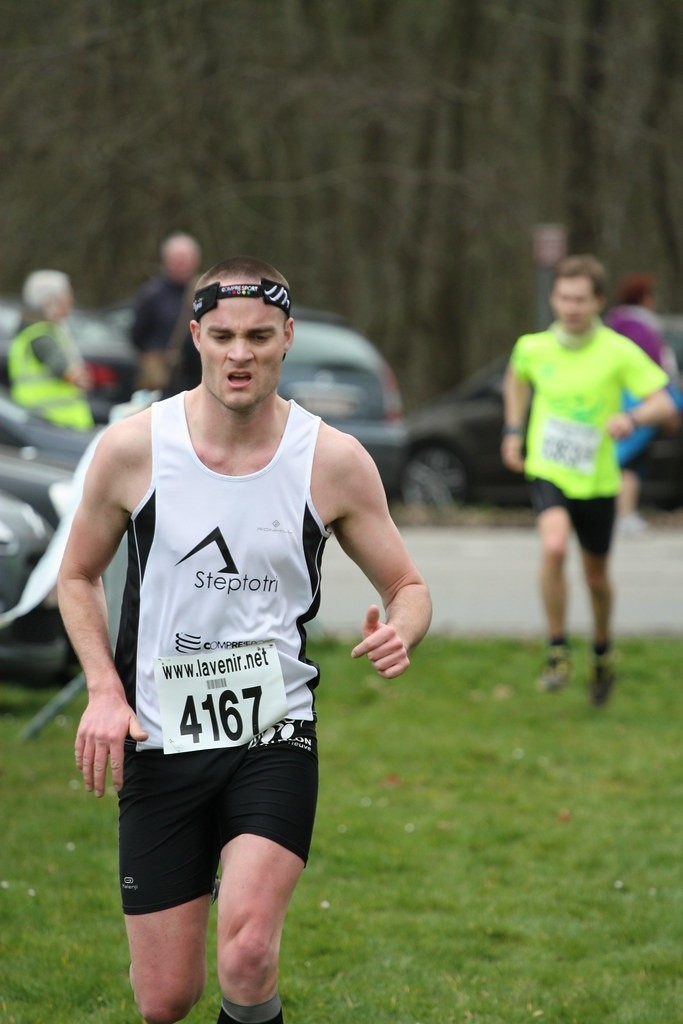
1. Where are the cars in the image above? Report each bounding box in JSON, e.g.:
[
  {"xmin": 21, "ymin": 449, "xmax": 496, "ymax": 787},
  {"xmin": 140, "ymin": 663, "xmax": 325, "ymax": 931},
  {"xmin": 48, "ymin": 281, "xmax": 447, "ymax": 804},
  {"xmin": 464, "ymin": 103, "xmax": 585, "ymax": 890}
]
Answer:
[
  {"xmin": 0, "ymin": 298, "xmax": 138, "ymax": 416},
  {"xmin": 101, "ymin": 301, "xmax": 408, "ymax": 503},
  {"xmin": 412, "ymin": 312, "xmax": 682, "ymax": 514},
  {"xmin": 0, "ymin": 396, "xmax": 104, "ymax": 687}
]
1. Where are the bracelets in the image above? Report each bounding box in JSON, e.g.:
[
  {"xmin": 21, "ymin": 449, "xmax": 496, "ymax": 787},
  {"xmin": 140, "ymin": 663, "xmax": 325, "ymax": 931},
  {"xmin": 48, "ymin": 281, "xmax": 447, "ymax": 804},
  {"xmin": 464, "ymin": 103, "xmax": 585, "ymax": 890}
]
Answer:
[
  {"xmin": 501, "ymin": 427, "xmax": 524, "ymax": 434},
  {"xmin": 627, "ymin": 411, "xmax": 639, "ymax": 429}
]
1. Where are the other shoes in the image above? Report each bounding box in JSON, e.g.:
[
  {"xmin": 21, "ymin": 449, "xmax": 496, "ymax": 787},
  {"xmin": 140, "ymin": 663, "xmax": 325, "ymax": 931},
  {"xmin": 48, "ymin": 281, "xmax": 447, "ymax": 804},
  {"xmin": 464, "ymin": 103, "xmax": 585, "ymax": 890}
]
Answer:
[
  {"xmin": 533, "ymin": 659, "xmax": 572, "ymax": 693},
  {"xmin": 589, "ymin": 666, "xmax": 615, "ymax": 705}
]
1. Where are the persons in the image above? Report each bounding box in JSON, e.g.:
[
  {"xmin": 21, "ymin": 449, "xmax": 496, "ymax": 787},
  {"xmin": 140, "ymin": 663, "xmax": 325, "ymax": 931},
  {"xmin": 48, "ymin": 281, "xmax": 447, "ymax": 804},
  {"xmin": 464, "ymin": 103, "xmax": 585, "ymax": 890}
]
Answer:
[
  {"xmin": 129, "ymin": 229, "xmax": 208, "ymax": 400},
  {"xmin": 55, "ymin": 257, "xmax": 433, "ymax": 1024},
  {"xmin": 603, "ymin": 273, "xmax": 678, "ymax": 530},
  {"xmin": 7, "ymin": 267, "xmax": 95, "ymax": 433},
  {"xmin": 499, "ymin": 253, "xmax": 675, "ymax": 704}
]
